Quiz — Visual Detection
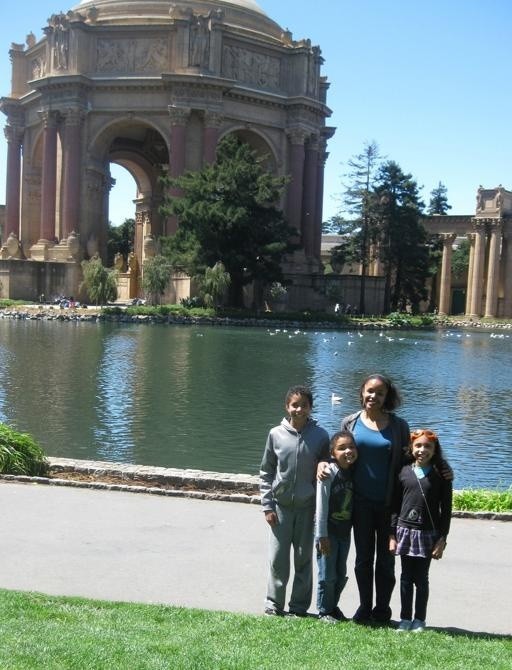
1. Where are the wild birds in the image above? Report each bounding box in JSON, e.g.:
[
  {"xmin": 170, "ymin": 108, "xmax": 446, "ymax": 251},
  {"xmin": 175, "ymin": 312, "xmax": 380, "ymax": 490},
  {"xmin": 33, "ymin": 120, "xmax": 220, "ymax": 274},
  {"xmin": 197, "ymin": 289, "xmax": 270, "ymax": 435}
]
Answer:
[
  {"xmin": 489, "ymin": 332, "xmax": 509, "ymax": 340},
  {"xmin": 332, "ymin": 392, "xmax": 344, "ymax": 401},
  {"xmin": 441, "ymin": 331, "xmax": 471, "ymax": 338},
  {"xmin": 265, "ymin": 327, "xmax": 419, "ymax": 355}
]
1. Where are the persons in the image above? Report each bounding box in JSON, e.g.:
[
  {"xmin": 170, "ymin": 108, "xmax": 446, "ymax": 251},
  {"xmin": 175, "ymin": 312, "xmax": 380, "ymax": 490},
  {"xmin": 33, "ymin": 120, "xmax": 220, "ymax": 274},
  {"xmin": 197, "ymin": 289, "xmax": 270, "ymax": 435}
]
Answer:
[
  {"xmin": 345, "ymin": 304, "xmax": 351, "ymax": 314},
  {"xmin": 312, "ymin": 431, "xmax": 358, "ymax": 623},
  {"xmin": 385, "ymin": 428, "xmax": 454, "ymax": 633},
  {"xmin": 351, "ymin": 305, "xmax": 359, "ymax": 315},
  {"xmin": 54, "ymin": 293, "xmax": 87, "ymax": 308},
  {"xmin": 312, "ymin": 373, "xmax": 454, "ymax": 627},
  {"xmin": 258, "ymin": 384, "xmax": 330, "ymax": 618},
  {"xmin": 40, "ymin": 292, "xmax": 46, "ymax": 303},
  {"xmin": 335, "ymin": 303, "xmax": 340, "ymax": 316},
  {"xmin": 131, "ymin": 297, "xmax": 142, "ymax": 305}
]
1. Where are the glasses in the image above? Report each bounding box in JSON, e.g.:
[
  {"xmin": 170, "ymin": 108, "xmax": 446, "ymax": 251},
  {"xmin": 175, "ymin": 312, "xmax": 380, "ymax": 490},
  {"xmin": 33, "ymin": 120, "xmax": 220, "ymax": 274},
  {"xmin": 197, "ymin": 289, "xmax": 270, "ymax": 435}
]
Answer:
[{"xmin": 412, "ymin": 429, "xmax": 436, "ymax": 442}]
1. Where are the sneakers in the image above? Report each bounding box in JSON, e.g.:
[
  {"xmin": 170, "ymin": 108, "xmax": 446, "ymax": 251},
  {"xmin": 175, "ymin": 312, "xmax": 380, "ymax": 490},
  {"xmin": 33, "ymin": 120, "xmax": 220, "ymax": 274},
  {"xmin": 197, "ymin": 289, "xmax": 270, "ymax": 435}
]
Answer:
[
  {"xmin": 353, "ymin": 606, "xmax": 392, "ymax": 626},
  {"xmin": 319, "ymin": 607, "xmax": 347, "ymax": 623},
  {"xmin": 265, "ymin": 609, "xmax": 309, "ymax": 616},
  {"xmin": 396, "ymin": 619, "xmax": 425, "ymax": 633}
]
